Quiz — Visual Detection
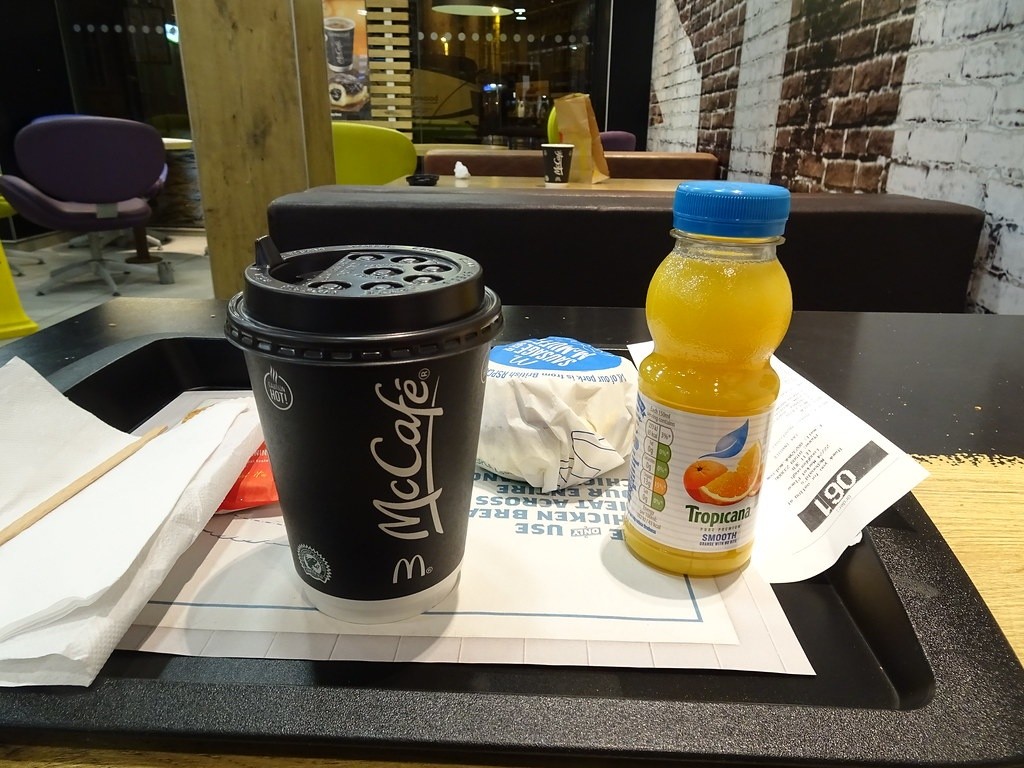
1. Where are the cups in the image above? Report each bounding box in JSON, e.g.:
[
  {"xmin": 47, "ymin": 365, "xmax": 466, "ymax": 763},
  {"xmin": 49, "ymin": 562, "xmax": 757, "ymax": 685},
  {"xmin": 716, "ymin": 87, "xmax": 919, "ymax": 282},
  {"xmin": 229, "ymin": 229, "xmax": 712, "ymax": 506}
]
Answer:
[
  {"xmin": 541, "ymin": 143, "xmax": 575, "ymax": 189},
  {"xmin": 224, "ymin": 236, "xmax": 505, "ymax": 625},
  {"xmin": 324, "ymin": 17, "xmax": 356, "ymax": 73}
]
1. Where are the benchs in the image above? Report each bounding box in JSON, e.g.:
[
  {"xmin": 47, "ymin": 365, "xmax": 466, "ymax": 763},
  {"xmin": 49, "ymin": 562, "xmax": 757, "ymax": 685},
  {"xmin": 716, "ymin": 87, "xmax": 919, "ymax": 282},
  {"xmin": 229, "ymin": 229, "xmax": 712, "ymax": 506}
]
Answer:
[{"xmin": 424, "ymin": 150, "xmax": 719, "ymax": 180}]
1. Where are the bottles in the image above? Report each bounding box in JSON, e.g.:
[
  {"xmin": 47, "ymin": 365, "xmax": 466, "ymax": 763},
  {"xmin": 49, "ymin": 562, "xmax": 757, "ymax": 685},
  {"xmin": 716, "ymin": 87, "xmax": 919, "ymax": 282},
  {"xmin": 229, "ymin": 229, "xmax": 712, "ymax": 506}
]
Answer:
[{"xmin": 622, "ymin": 182, "xmax": 794, "ymax": 578}]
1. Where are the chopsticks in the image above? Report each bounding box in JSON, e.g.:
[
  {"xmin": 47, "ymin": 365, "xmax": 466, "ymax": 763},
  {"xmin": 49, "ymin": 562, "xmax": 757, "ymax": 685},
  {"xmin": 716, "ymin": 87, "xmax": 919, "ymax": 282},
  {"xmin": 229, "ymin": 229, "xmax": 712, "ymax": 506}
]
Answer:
[{"xmin": 0, "ymin": 423, "xmax": 169, "ymax": 544}]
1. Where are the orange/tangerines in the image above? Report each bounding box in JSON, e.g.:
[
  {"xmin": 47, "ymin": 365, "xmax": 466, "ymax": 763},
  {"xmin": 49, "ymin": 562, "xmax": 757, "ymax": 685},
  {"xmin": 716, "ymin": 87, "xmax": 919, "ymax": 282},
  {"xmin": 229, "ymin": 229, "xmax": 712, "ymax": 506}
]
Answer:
[{"xmin": 683, "ymin": 440, "xmax": 763, "ymax": 506}]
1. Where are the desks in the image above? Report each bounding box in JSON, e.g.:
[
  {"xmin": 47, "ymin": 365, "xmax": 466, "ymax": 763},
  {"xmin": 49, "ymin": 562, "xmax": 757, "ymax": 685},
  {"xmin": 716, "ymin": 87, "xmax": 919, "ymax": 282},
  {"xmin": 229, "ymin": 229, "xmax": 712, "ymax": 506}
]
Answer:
[{"xmin": 0, "ymin": 307, "xmax": 1024, "ymax": 768}]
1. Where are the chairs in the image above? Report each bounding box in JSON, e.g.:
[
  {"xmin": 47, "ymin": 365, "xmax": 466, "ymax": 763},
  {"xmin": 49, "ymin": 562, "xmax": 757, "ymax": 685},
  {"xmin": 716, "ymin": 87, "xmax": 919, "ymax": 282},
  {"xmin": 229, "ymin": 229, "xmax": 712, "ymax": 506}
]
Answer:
[
  {"xmin": 329, "ymin": 122, "xmax": 417, "ymax": 186},
  {"xmin": 0, "ymin": 115, "xmax": 170, "ymax": 297},
  {"xmin": 599, "ymin": 131, "xmax": 636, "ymax": 152}
]
